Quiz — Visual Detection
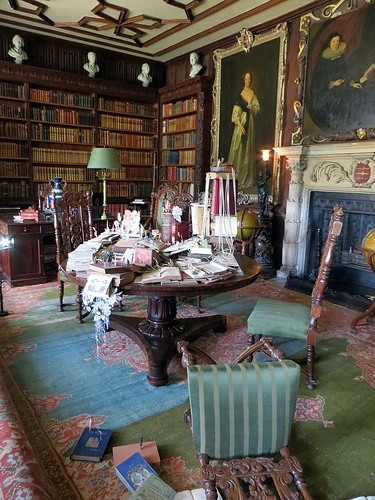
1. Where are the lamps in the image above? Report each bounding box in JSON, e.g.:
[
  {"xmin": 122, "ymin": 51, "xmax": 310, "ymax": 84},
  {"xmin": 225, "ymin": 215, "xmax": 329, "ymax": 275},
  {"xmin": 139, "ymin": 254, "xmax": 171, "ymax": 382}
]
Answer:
[
  {"xmin": 88, "ymin": 148, "xmax": 121, "ymax": 221},
  {"xmin": 261, "ymin": 150, "xmax": 271, "ymax": 182}
]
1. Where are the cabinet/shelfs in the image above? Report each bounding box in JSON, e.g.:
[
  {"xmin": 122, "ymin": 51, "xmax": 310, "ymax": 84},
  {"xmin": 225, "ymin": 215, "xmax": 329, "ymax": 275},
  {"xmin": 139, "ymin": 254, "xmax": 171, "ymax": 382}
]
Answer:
[{"xmin": 0, "ymin": 62, "xmax": 206, "ymax": 216}]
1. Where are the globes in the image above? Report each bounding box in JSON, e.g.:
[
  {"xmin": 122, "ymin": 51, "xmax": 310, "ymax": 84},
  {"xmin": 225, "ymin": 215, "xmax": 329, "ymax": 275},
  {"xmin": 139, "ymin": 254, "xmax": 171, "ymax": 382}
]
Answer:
[
  {"xmin": 352, "ymin": 229, "xmax": 375, "ymax": 333},
  {"xmin": 237, "ymin": 207, "xmax": 265, "ymax": 257}
]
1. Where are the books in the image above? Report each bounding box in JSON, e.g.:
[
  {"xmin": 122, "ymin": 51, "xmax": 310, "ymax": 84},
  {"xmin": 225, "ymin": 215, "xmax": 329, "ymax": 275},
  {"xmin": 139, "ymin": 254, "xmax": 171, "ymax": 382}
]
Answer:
[
  {"xmin": 106, "ymin": 203, "xmax": 137, "ymax": 218},
  {"xmin": 0, "ymin": 82, "xmax": 197, "ymax": 200},
  {"xmin": 113, "ymin": 441, "xmax": 161, "ymax": 469},
  {"xmin": 19, "ymin": 207, "xmax": 45, "ymax": 220},
  {"xmin": 115, "ymin": 452, "xmax": 223, "ymax": 500},
  {"xmin": 65, "ymin": 233, "xmax": 244, "ymax": 298},
  {"xmin": 70, "ymin": 427, "xmax": 113, "ymax": 463}
]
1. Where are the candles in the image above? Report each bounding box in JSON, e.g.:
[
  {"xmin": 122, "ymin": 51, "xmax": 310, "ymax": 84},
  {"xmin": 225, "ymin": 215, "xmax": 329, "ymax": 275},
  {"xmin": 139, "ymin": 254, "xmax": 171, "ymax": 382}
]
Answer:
[{"xmin": 169, "ymin": 167, "xmax": 174, "ymax": 183}]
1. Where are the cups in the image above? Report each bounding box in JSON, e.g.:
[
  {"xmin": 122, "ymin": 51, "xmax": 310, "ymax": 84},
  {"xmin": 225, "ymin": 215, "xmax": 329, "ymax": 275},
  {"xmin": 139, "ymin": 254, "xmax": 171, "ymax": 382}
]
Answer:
[{"xmin": 104, "ymin": 246, "xmax": 116, "ymax": 269}]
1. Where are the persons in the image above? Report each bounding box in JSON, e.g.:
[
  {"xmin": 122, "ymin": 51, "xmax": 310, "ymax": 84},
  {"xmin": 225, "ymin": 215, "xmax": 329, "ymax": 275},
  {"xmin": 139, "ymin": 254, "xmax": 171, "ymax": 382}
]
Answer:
[
  {"xmin": 137, "ymin": 63, "xmax": 152, "ymax": 82},
  {"xmin": 189, "ymin": 52, "xmax": 202, "ymax": 77},
  {"xmin": 8, "ymin": 35, "xmax": 28, "ymax": 59},
  {"xmin": 83, "ymin": 51, "xmax": 100, "ymax": 73}
]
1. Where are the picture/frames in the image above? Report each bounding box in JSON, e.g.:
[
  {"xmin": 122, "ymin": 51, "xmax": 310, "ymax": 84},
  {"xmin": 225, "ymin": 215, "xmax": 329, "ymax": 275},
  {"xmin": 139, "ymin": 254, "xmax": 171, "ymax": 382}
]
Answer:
[
  {"xmin": 291, "ymin": 1, "xmax": 375, "ymax": 144},
  {"xmin": 210, "ymin": 22, "xmax": 287, "ymax": 208}
]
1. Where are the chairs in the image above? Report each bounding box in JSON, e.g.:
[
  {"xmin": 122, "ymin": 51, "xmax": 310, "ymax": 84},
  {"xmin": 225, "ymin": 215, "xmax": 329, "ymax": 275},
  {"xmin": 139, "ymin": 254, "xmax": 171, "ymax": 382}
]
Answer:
[
  {"xmin": 247, "ymin": 206, "xmax": 342, "ymax": 392},
  {"xmin": 50, "ymin": 189, "xmax": 124, "ymax": 323},
  {"xmin": 178, "ymin": 336, "xmax": 312, "ymax": 500},
  {"xmin": 144, "ymin": 183, "xmax": 179, "ymax": 232}
]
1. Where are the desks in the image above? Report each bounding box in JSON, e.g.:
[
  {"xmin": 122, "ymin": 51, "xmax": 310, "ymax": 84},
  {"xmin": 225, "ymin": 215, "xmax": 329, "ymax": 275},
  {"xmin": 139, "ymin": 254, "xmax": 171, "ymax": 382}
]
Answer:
[
  {"xmin": 65, "ymin": 253, "xmax": 260, "ymax": 387},
  {"xmin": 1, "ymin": 215, "xmax": 117, "ymax": 288}
]
